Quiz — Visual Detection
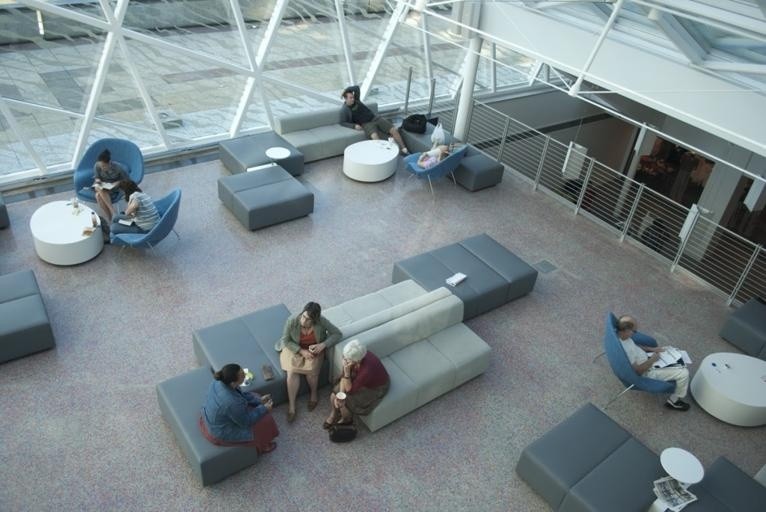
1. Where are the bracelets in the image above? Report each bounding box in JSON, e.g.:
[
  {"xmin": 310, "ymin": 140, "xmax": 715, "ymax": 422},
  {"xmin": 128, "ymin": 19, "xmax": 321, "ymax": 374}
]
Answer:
[{"xmin": 344, "ymin": 375, "xmax": 350, "ymax": 379}]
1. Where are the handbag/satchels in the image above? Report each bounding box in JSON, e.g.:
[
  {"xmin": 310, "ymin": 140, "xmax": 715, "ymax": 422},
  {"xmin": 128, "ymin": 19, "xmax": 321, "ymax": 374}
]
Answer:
[
  {"xmin": 403, "ymin": 114, "xmax": 427, "ymax": 133},
  {"xmin": 329, "ymin": 426, "xmax": 357, "ymax": 443}
]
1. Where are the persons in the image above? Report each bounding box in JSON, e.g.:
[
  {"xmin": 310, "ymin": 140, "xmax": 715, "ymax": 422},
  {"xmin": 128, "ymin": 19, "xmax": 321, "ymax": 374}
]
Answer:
[
  {"xmin": 639, "ymin": 218, "xmax": 671, "ymax": 253},
  {"xmin": 197, "ymin": 363, "xmax": 280, "ymax": 458},
  {"xmin": 322, "ymin": 338, "xmax": 391, "ymax": 431},
  {"xmin": 668, "ymin": 152, "xmax": 698, "ymax": 206},
  {"xmin": 102, "ymin": 178, "xmax": 161, "ymax": 244},
  {"xmin": 92, "ymin": 149, "xmax": 130, "ymax": 223},
  {"xmin": 272, "ymin": 301, "xmax": 343, "ymax": 422},
  {"xmin": 572, "ymin": 179, "xmax": 595, "ymax": 211},
  {"xmin": 339, "ymin": 85, "xmax": 412, "ymax": 158},
  {"xmin": 615, "ymin": 314, "xmax": 690, "ymax": 410}
]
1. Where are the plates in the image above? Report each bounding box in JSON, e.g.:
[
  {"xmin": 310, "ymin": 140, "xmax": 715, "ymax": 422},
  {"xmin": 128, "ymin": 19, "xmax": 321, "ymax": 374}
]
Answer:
[
  {"xmin": 266, "ymin": 147, "xmax": 290, "ymax": 159},
  {"xmin": 660, "ymin": 445, "xmax": 707, "ymax": 484}
]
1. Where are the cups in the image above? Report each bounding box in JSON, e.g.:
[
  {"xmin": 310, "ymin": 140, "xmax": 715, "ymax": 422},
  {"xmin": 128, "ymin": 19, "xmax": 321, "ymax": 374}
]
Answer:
[
  {"xmin": 71, "ymin": 197, "xmax": 78, "ymax": 208},
  {"xmin": 337, "ymin": 392, "xmax": 348, "ymax": 409}
]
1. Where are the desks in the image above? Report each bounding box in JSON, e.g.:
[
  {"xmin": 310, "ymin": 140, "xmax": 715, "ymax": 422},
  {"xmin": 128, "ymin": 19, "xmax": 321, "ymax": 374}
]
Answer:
[
  {"xmin": 343, "ymin": 138, "xmax": 400, "ymax": 183},
  {"xmin": 690, "ymin": 352, "xmax": 766, "ymax": 427},
  {"xmin": 29, "ymin": 201, "xmax": 105, "ymax": 266}
]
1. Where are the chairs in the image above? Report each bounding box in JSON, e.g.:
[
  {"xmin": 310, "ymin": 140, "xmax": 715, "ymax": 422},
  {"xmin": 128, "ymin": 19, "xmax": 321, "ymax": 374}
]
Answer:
[
  {"xmin": 110, "ymin": 188, "xmax": 181, "ymax": 264},
  {"xmin": 592, "ymin": 310, "xmax": 676, "ymax": 410},
  {"xmin": 74, "ymin": 137, "xmax": 145, "ymax": 212},
  {"xmin": 401, "ymin": 144, "xmax": 470, "ymax": 199}
]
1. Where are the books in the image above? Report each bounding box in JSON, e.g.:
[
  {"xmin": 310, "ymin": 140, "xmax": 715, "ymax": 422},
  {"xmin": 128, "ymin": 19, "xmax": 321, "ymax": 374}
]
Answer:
[
  {"xmin": 117, "ymin": 213, "xmax": 136, "ymax": 226},
  {"xmin": 90, "ymin": 181, "xmax": 116, "ymax": 191},
  {"xmin": 650, "ymin": 345, "xmax": 683, "ymax": 369}
]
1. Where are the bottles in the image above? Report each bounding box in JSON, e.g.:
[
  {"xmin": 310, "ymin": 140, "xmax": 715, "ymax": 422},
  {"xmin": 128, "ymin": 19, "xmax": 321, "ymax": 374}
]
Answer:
[
  {"xmin": 90, "ymin": 212, "xmax": 98, "ymax": 227},
  {"xmin": 448, "ymin": 133, "xmax": 454, "ymax": 152}
]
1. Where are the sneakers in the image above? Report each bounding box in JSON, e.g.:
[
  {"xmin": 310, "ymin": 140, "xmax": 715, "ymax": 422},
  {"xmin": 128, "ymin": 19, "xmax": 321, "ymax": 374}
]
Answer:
[
  {"xmin": 400, "ymin": 148, "xmax": 411, "ymax": 156},
  {"xmin": 262, "ymin": 442, "xmax": 276, "ymax": 452},
  {"xmin": 664, "ymin": 397, "xmax": 689, "ymax": 411}
]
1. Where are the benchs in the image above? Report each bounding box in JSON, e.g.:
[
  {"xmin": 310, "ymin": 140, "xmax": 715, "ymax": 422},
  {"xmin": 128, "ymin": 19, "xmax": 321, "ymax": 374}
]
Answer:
[
  {"xmin": 558, "ymin": 437, "xmax": 670, "ymax": 512},
  {"xmin": 157, "ymin": 365, "xmax": 257, "ymax": 486},
  {"xmin": 218, "ymin": 130, "xmax": 304, "ymax": 175},
  {"xmin": 719, "ymin": 297, "xmax": 766, "ymax": 357},
  {"xmin": 192, "ymin": 304, "xmax": 329, "ymax": 408},
  {"xmin": 0, "ymin": 192, "xmax": 9, "ymax": 230},
  {"xmin": 515, "ymin": 402, "xmax": 632, "ymax": 512},
  {"xmin": 758, "ymin": 342, "xmax": 766, "ymax": 361},
  {"xmin": 396, "ymin": 121, "xmax": 504, "ymax": 193},
  {"xmin": 391, "ymin": 232, "xmax": 538, "ymax": 322},
  {"xmin": 698, "ymin": 456, "xmax": 765, "ymax": 512},
  {"xmin": 217, "ymin": 165, "xmax": 314, "ymax": 232},
  {"xmin": 0, "ymin": 269, "xmax": 54, "ymax": 365},
  {"xmin": 664, "ymin": 484, "xmax": 766, "ymax": 512}
]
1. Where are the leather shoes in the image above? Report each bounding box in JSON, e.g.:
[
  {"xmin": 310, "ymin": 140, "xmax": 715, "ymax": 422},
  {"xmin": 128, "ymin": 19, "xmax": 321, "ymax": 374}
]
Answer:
[
  {"xmin": 324, "ymin": 414, "xmax": 339, "ymax": 429},
  {"xmin": 333, "ymin": 416, "xmax": 353, "ymax": 425},
  {"xmin": 308, "ymin": 401, "xmax": 317, "ymax": 412},
  {"xmin": 287, "ymin": 408, "xmax": 295, "ymax": 422}
]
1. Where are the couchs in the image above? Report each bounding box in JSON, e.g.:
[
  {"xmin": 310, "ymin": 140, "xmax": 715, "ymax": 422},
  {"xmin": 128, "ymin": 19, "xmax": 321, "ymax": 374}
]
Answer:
[
  {"xmin": 274, "ymin": 102, "xmax": 392, "ymax": 163},
  {"xmin": 320, "ymin": 279, "xmax": 453, "ymax": 385},
  {"xmin": 332, "ymin": 295, "xmax": 492, "ymax": 433}
]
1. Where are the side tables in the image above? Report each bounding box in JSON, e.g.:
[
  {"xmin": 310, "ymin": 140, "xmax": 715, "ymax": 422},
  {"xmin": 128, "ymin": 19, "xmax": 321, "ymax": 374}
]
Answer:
[
  {"xmin": 647, "ymin": 447, "xmax": 705, "ymax": 497},
  {"xmin": 246, "ymin": 146, "xmax": 291, "ymax": 173}
]
